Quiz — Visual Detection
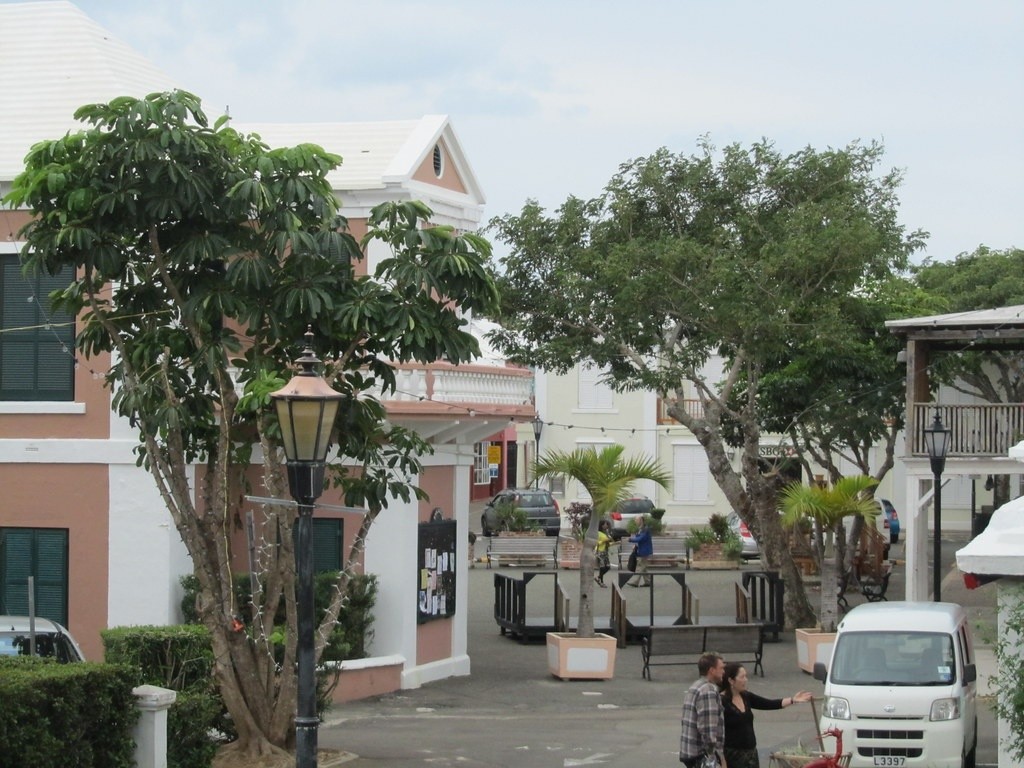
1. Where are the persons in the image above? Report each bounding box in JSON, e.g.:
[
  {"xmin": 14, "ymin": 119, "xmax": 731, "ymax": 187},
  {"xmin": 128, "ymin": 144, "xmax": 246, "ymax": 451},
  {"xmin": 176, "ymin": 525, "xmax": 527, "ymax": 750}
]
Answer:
[
  {"xmin": 679, "ymin": 655, "xmax": 726, "ymax": 768},
  {"xmin": 721, "ymin": 662, "xmax": 812, "ymax": 768},
  {"xmin": 595, "ymin": 520, "xmax": 621, "ymax": 588},
  {"xmin": 626, "ymin": 516, "xmax": 652, "ymax": 587}
]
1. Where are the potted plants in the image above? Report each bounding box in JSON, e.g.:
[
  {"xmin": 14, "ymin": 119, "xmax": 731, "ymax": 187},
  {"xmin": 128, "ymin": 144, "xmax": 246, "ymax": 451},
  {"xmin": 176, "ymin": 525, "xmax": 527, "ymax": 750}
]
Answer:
[
  {"xmin": 687, "ymin": 513, "xmax": 742, "ymax": 562},
  {"xmin": 775, "ymin": 474, "xmax": 885, "ymax": 676},
  {"xmin": 521, "ymin": 441, "xmax": 677, "ymax": 680}
]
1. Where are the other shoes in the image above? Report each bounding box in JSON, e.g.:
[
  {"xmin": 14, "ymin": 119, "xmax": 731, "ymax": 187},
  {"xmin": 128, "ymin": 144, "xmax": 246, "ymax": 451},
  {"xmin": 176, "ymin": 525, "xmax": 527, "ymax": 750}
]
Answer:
[
  {"xmin": 594, "ymin": 576, "xmax": 602, "ymax": 586},
  {"xmin": 600, "ymin": 583, "xmax": 607, "ymax": 588},
  {"xmin": 627, "ymin": 581, "xmax": 638, "ymax": 587},
  {"xmin": 640, "ymin": 583, "xmax": 650, "ymax": 587}
]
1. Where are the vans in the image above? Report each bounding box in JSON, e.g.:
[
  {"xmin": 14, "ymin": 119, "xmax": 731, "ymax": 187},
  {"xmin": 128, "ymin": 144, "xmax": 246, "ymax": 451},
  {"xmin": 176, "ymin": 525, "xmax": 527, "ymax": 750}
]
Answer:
[
  {"xmin": 813, "ymin": 601, "xmax": 979, "ymax": 768},
  {"xmin": 808, "ymin": 498, "xmax": 892, "ymax": 560}
]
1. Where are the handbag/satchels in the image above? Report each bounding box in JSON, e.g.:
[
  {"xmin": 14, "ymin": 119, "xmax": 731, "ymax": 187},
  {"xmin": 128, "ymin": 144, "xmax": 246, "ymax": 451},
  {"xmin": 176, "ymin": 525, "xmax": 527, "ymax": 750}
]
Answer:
[{"xmin": 627, "ymin": 548, "xmax": 637, "ymax": 572}]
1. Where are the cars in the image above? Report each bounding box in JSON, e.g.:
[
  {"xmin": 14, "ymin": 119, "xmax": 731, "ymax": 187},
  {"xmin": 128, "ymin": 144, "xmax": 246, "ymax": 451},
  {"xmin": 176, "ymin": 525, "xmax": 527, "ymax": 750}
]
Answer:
[
  {"xmin": 724, "ymin": 510, "xmax": 760, "ymax": 558},
  {"xmin": 0, "ymin": 614, "xmax": 87, "ymax": 666},
  {"xmin": 881, "ymin": 498, "xmax": 900, "ymax": 543},
  {"xmin": 603, "ymin": 496, "xmax": 655, "ymax": 540}
]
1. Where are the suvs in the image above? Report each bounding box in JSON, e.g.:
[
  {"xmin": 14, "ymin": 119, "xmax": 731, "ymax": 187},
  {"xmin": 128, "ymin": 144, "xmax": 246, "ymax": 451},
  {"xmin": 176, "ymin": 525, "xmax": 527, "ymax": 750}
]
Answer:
[{"xmin": 481, "ymin": 487, "xmax": 561, "ymax": 537}]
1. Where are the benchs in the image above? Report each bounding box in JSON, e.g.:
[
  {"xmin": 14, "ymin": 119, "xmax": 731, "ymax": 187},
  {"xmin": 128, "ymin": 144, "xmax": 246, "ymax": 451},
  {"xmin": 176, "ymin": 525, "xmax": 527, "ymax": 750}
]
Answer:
[
  {"xmin": 618, "ymin": 537, "xmax": 690, "ymax": 571},
  {"xmin": 639, "ymin": 622, "xmax": 766, "ymax": 680},
  {"xmin": 486, "ymin": 537, "xmax": 560, "ymax": 570}
]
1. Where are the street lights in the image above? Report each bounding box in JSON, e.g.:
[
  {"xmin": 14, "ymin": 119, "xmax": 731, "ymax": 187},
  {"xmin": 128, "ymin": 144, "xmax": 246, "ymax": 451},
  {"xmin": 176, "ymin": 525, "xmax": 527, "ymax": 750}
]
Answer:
[
  {"xmin": 269, "ymin": 322, "xmax": 347, "ymax": 768},
  {"xmin": 531, "ymin": 415, "xmax": 543, "ymax": 489},
  {"xmin": 922, "ymin": 403, "xmax": 953, "ymax": 601}
]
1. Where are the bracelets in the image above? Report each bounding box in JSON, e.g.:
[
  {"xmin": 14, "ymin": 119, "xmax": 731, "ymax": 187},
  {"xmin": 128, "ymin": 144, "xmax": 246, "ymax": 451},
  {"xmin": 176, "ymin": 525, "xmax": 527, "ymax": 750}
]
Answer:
[{"xmin": 791, "ymin": 697, "xmax": 793, "ymax": 704}]
